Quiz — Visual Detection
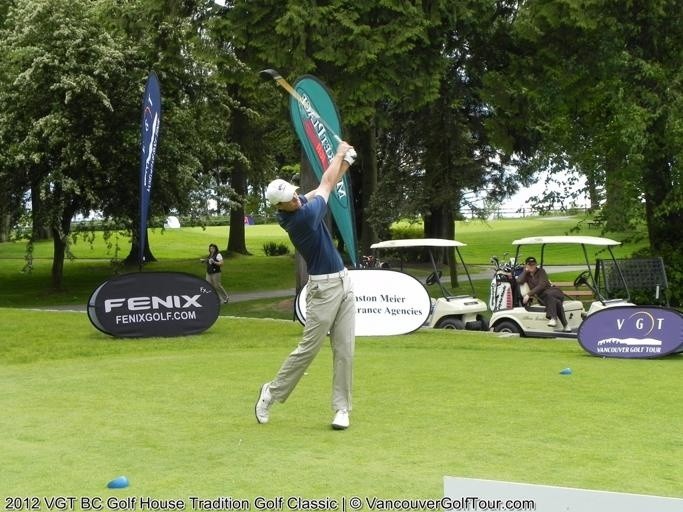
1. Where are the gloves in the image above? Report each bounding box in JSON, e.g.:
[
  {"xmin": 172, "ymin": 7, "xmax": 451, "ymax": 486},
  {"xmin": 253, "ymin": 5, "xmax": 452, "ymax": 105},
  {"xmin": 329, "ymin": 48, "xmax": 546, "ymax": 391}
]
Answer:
[{"xmin": 343, "ymin": 148, "xmax": 357, "ymax": 166}]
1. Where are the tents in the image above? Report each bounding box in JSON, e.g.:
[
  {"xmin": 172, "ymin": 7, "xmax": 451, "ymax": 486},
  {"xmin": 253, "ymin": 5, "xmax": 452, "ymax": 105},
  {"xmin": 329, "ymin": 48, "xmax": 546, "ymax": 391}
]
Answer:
[
  {"xmin": 163, "ymin": 215, "xmax": 180, "ymax": 228},
  {"xmin": 244, "ymin": 215, "xmax": 255, "ymax": 225}
]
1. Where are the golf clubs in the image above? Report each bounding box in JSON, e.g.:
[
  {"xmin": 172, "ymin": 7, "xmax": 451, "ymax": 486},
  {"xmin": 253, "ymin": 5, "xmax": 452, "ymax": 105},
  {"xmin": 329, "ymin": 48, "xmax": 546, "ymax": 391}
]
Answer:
[
  {"xmin": 489, "ymin": 252, "xmax": 521, "ymax": 281},
  {"xmin": 256, "ymin": 69, "xmax": 342, "ymax": 143}
]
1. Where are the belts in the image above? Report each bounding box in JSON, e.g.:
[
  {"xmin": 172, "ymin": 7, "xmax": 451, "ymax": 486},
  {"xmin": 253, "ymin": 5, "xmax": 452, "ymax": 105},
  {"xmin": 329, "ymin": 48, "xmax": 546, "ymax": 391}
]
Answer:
[{"xmin": 308, "ymin": 267, "xmax": 349, "ymax": 281}]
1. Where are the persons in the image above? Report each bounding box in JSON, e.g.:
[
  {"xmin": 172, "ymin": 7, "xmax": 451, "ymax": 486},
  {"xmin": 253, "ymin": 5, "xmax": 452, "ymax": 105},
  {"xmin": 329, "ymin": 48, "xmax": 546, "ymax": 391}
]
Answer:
[
  {"xmin": 199, "ymin": 243, "xmax": 229, "ymax": 304},
  {"xmin": 516, "ymin": 256, "xmax": 572, "ymax": 333},
  {"xmin": 18, "ymin": 238, "xmax": 33, "ymax": 275},
  {"xmin": 254, "ymin": 141, "xmax": 357, "ymax": 431},
  {"xmin": 351, "ymin": 248, "xmax": 388, "ymax": 269}
]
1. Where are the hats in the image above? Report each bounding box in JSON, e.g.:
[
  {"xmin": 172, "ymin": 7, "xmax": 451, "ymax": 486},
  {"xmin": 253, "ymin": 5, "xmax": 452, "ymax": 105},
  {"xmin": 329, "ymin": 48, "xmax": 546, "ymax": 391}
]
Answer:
[
  {"xmin": 266, "ymin": 179, "xmax": 300, "ymax": 205},
  {"xmin": 525, "ymin": 257, "xmax": 536, "ymax": 263}
]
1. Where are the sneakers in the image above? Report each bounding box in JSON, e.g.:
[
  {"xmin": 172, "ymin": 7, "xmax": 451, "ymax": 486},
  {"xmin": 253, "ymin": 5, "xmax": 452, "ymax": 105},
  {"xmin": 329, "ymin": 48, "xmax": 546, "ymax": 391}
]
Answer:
[
  {"xmin": 255, "ymin": 380, "xmax": 274, "ymax": 425},
  {"xmin": 547, "ymin": 318, "xmax": 557, "ymax": 327},
  {"xmin": 564, "ymin": 325, "xmax": 572, "ymax": 332},
  {"xmin": 331, "ymin": 409, "xmax": 349, "ymax": 430}
]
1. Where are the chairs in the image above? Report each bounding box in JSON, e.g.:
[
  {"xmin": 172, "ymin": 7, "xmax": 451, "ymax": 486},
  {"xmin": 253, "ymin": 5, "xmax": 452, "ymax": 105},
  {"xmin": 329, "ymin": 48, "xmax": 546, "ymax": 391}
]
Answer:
[{"xmin": 517, "ymin": 281, "xmax": 583, "ymax": 312}]
